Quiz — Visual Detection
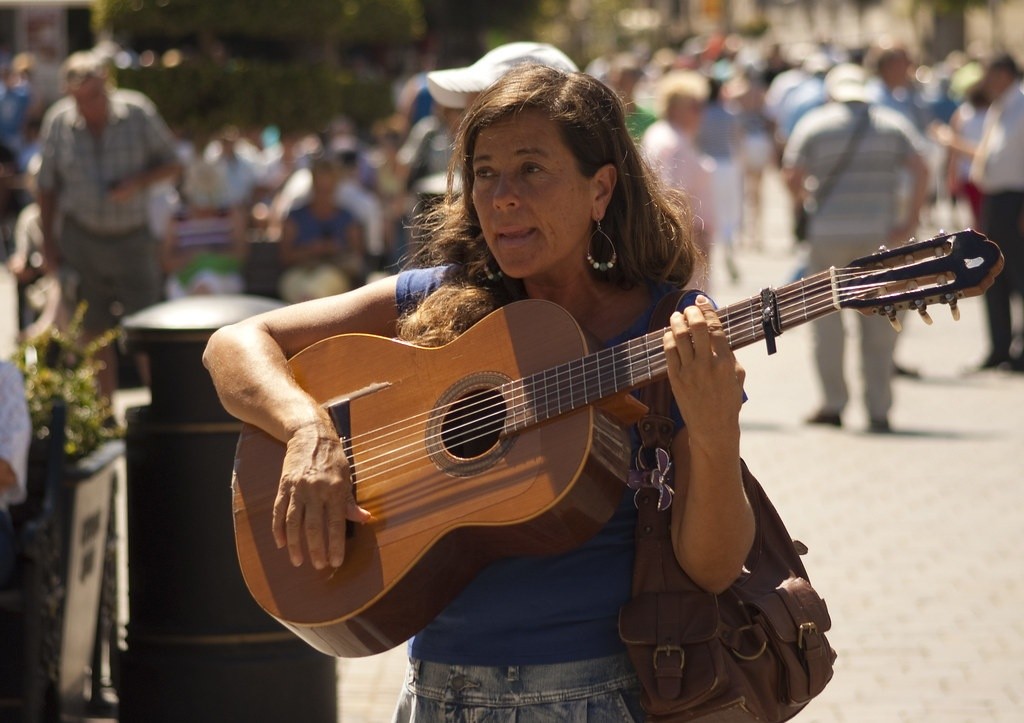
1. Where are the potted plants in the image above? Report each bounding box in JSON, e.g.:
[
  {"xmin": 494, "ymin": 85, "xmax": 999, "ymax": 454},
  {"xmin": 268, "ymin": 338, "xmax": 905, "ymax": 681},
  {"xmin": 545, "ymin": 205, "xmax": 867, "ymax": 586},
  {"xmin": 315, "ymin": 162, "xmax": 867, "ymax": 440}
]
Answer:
[{"xmin": 0, "ymin": 296, "xmax": 131, "ymax": 723}]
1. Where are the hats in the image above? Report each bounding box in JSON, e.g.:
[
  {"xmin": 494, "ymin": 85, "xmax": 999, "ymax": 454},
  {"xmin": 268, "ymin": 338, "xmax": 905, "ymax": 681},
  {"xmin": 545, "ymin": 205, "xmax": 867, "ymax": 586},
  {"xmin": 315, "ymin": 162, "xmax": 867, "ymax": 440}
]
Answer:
[{"xmin": 427, "ymin": 41, "xmax": 579, "ymax": 109}]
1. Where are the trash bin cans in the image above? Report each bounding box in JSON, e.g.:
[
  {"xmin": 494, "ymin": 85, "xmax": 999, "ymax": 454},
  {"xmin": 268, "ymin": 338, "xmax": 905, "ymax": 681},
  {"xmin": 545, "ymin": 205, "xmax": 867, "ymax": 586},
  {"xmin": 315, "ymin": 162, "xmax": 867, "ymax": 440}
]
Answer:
[{"xmin": 117, "ymin": 293, "xmax": 337, "ymax": 723}]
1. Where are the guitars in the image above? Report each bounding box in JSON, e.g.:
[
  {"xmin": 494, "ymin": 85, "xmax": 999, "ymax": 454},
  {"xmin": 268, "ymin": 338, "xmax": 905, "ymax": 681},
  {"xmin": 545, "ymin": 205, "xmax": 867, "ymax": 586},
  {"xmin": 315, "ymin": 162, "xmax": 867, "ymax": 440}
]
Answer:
[{"xmin": 231, "ymin": 229, "xmax": 1003, "ymax": 659}]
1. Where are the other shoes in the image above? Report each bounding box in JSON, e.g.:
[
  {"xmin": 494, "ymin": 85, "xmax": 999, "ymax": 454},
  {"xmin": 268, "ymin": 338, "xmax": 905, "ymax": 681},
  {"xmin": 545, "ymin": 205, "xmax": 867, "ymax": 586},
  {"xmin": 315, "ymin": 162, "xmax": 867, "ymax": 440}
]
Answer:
[
  {"xmin": 981, "ymin": 348, "xmax": 1024, "ymax": 372},
  {"xmin": 867, "ymin": 418, "xmax": 888, "ymax": 432},
  {"xmin": 804, "ymin": 407, "xmax": 840, "ymax": 426}
]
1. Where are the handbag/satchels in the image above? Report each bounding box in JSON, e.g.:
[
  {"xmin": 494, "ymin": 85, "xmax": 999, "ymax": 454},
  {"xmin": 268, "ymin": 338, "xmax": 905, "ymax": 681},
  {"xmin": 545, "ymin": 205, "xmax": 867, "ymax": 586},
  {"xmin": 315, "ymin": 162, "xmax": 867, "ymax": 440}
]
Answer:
[{"xmin": 620, "ymin": 289, "xmax": 836, "ymax": 723}]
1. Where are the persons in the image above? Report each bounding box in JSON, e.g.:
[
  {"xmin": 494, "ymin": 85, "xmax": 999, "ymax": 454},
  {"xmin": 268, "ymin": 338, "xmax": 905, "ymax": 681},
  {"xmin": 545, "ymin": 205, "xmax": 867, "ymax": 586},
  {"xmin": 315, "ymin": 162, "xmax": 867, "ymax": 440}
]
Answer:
[
  {"xmin": 201, "ymin": 65, "xmax": 838, "ymax": 723},
  {"xmin": 0, "ymin": 37, "xmax": 1024, "ymax": 435},
  {"xmin": 0, "ymin": 363, "xmax": 31, "ymax": 511}
]
